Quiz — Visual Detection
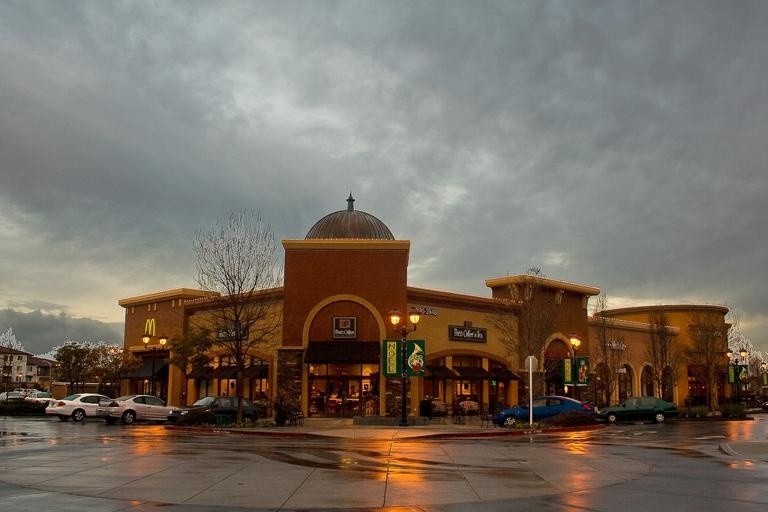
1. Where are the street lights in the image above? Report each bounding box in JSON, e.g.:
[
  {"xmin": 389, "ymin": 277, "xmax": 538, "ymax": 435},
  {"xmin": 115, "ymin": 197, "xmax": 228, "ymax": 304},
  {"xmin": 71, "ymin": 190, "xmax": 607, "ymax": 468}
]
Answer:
[
  {"xmin": 388, "ymin": 306, "xmax": 421, "ymax": 427},
  {"xmin": 726, "ymin": 349, "xmax": 746, "ymax": 403},
  {"xmin": 570, "ymin": 337, "xmax": 581, "ymax": 400},
  {"xmin": 18, "ymin": 373, "xmax": 22, "ymax": 399},
  {"xmin": 141, "ymin": 331, "xmax": 168, "ymax": 394}
]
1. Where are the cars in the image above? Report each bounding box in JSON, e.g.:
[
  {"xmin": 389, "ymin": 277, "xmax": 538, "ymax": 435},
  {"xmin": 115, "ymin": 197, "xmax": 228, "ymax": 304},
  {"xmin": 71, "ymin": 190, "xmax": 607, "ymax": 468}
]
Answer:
[
  {"xmin": 167, "ymin": 395, "xmax": 267, "ymax": 425},
  {"xmin": 46, "ymin": 393, "xmax": 112, "ymax": 422},
  {"xmin": 0, "ymin": 387, "xmax": 55, "ymax": 403},
  {"xmin": 600, "ymin": 396, "xmax": 677, "ymax": 423},
  {"xmin": 96, "ymin": 395, "xmax": 180, "ymax": 423},
  {"xmin": 493, "ymin": 395, "xmax": 593, "ymax": 428}
]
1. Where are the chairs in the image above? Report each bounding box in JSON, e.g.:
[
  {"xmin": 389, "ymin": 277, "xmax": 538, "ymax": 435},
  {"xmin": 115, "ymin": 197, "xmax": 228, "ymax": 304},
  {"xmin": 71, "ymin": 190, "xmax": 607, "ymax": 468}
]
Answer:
[{"xmin": 480, "ymin": 408, "xmax": 497, "ymax": 428}]
